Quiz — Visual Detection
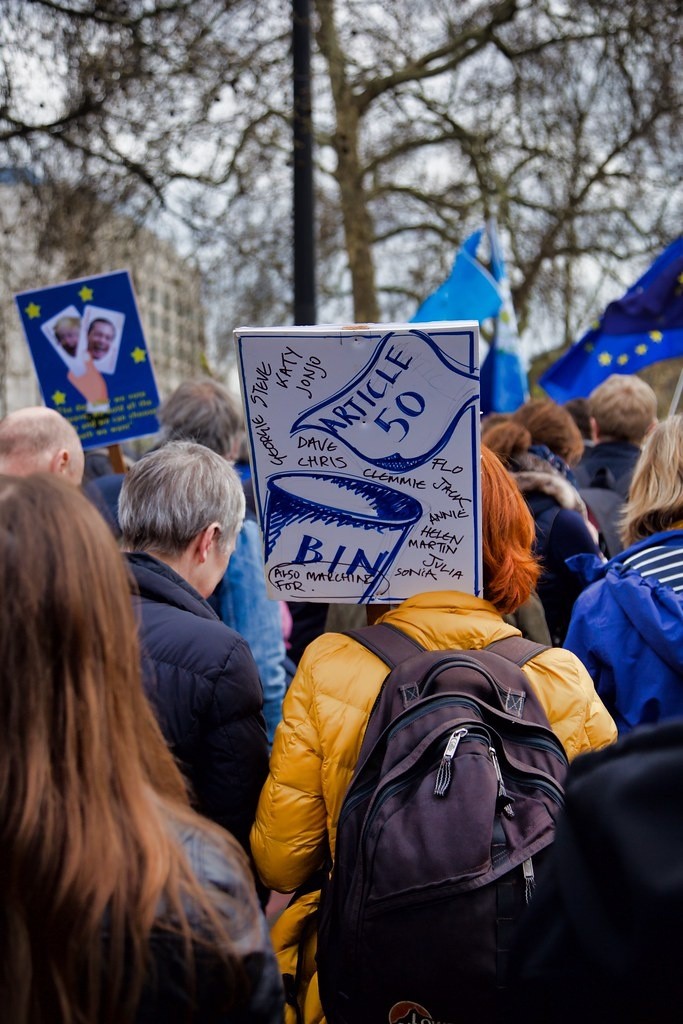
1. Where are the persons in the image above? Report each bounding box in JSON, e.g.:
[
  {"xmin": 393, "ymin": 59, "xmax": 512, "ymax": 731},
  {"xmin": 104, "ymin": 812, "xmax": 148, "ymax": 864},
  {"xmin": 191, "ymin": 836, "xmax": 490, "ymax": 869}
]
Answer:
[
  {"xmin": 0, "ymin": 471, "xmax": 284, "ymax": 1024},
  {"xmin": 0, "ymin": 379, "xmax": 327, "ymax": 750},
  {"xmin": 251, "ymin": 443, "xmax": 618, "ymax": 1024},
  {"xmin": 116, "ymin": 440, "xmax": 271, "ymax": 916},
  {"xmin": 480, "ymin": 375, "xmax": 659, "ymax": 645},
  {"xmin": 87, "ymin": 319, "xmax": 115, "ymax": 359},
  {"xmin": 565, "ymin": 414, "xmax": 683, "ymax": 735},
  {"xmin": 54, "ymin": 317, "xmax": 81, "ymax": 356},
  {"xmin": 495, "ymin": 719, "xmax": 683, "ymax": 1024},
  {"xmin": 326, "ymin": 583, "xmax": 552, "ymax": 646}
]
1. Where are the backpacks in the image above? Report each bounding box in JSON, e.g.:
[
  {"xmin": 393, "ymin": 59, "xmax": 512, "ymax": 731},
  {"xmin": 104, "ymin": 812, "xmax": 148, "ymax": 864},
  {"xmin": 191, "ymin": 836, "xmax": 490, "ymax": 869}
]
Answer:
[{"xmin": 326, "ymin": 623, "xmax": 568, "ymax": 1024}]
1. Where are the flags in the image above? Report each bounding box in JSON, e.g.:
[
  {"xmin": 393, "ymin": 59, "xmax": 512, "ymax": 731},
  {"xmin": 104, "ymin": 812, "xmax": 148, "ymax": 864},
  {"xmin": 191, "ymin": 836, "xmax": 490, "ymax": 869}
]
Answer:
[
  {"xmin": 410, "ymin": 229, "xmax": 502, "ymax": 327},
  {"xmin": 478, "ymin": 224, "xmax": 530, "ymax": 413},
  {"xmin": 601, "ymin": 231, "xmax": 683, "ymax": 332},
  {"xmin": 537, "ymin": 316, "xmax": 683, "ymax": 404}
]
